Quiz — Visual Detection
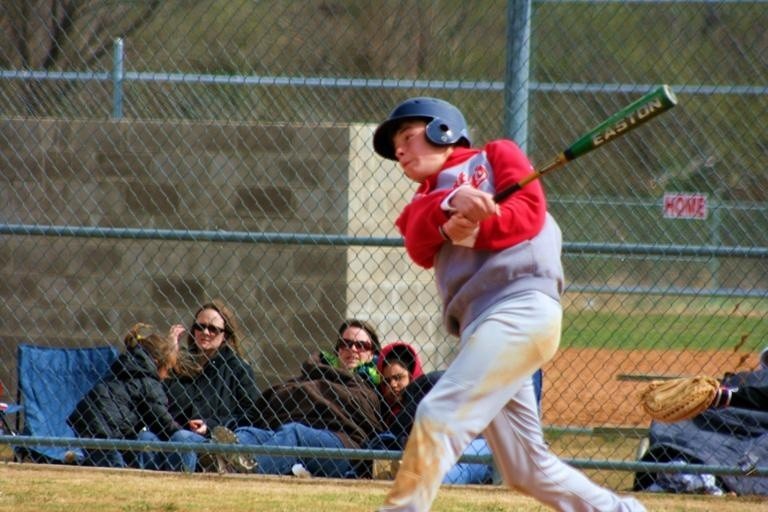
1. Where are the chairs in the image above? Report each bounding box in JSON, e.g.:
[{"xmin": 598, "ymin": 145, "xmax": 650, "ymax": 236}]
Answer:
[{"xmin": 2, "ymin": 345, "xmax": 119, "ymax": 464}]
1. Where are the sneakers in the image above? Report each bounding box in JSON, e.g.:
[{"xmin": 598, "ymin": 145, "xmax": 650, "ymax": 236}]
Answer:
[{"xmin": 210, "ymin": 424, "xmax": 259, "ymax": 474}]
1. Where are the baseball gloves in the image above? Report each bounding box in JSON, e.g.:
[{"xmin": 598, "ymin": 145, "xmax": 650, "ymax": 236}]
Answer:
[{"xmin": 638, "ymin": 374, "xmax": 718, "ymax": 423}]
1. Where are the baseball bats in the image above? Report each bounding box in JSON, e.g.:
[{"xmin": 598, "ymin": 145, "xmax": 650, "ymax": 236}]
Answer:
[{"xmin": 437, "ymin": 84, "xmax": 678, "ymax": 241}]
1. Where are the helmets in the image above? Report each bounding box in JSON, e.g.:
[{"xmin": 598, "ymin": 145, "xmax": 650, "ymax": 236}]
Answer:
[{"xmin": 370, "ymin": 94, "xmax": 475, "ymax": 162}]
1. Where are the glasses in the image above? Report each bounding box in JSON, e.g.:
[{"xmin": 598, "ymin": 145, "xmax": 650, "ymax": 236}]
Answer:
[
  {"xmin": 193, "ymin": 322, "xmax": 227, "ymax": 334},
  {"xmin": 338, "ymin": 337, "xmax": 374, "ymax": 351}
]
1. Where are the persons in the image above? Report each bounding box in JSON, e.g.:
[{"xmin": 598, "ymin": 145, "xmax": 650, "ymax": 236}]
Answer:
[
  {"xmin": 233, "ymin": 317, "xmax": 381, "ymax": 480},
  {"xmin": 372, "ymin": 342, "xmax": 501, "ymax": 484},
  {"xmin": 65, "ymin": 324, "xmax": 186, "ymax": 470},
  {"xmin": 128, "ymin": 298, "xmax": 256, "ymax": 472},
  {"xmin": 636, "ymin": 374, "xmax": 766, "ymax": 424},
  {"xmin": 371, "ymin": 94, "xmax": 645, "ymax": 512}
]
